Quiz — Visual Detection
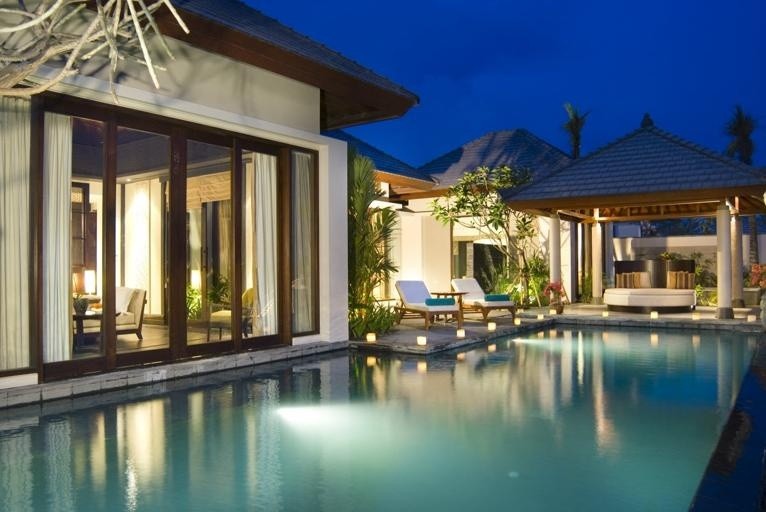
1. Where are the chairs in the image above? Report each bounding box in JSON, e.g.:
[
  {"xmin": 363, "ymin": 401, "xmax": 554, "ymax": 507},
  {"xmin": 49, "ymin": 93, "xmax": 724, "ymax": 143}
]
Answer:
[
  {"xmin": 207, "ymin": 287, "xmax": 255, "ymax": 341},
  {"xmin": 394, "ymin": 276, "xmax": 518, "ymax": 329},
  {"xmin": 72, "ymin": 286, "xmax": 147, "ymax": 342}
]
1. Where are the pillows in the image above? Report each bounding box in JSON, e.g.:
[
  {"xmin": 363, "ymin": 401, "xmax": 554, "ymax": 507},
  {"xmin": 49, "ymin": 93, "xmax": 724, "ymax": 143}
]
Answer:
[{"xmin": 114, "ymin": 288, "xmax": 134, "ymax": 314}]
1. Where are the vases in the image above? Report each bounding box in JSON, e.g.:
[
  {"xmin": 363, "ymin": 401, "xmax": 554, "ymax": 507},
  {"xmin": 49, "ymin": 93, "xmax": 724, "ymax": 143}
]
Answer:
[
  {"xmin": 551, "ymin": 302, "xmax": 565, "ymax": 313},
  {"xmin": 72, "ymin": 293, "xmax": 89, "ymax": 313}
]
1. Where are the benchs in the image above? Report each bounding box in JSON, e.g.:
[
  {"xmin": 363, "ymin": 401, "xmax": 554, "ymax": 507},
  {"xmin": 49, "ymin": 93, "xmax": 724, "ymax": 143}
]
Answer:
[{"xmin": 605, "ymin": 286, "xmax": 697, "ymax": 311}]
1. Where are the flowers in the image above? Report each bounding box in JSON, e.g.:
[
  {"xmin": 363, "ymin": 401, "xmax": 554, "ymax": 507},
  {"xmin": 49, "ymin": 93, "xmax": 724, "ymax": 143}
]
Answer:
[
  {"xmin": 751, "ymin": 264, "xmax": 766, "ymax": 287},
  {"xmin": 544, "ymin": 282, "xmax": 567, "ymax": 306}
]
